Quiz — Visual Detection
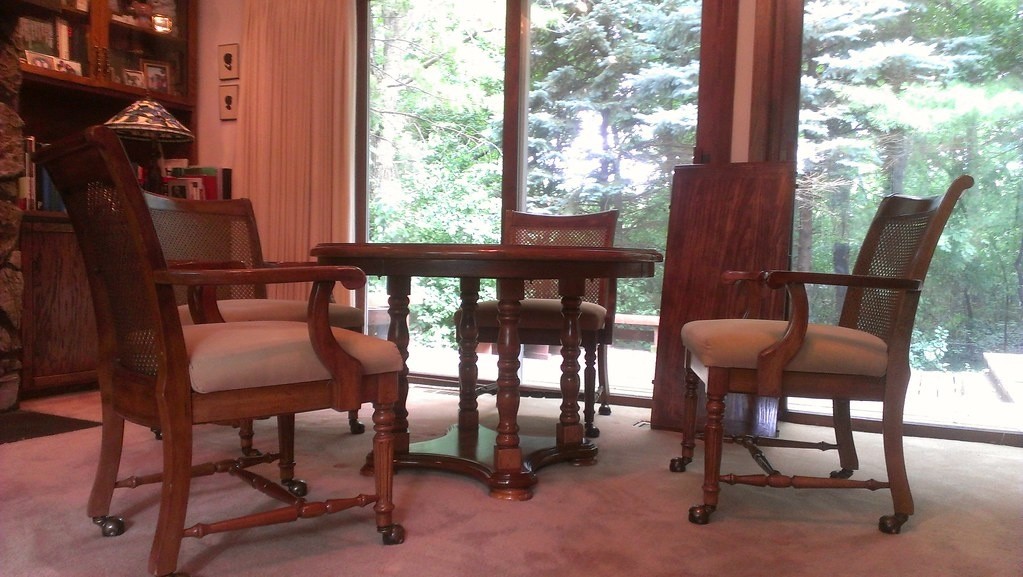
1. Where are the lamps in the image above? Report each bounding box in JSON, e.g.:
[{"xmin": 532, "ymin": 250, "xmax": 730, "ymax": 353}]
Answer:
[{"xmin": 103, "ymin": 91, "xmax": 196, "ymax": 193}]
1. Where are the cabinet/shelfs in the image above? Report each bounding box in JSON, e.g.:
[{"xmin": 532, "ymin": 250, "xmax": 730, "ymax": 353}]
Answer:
[{"xmin": 12, "ymin": 0, "xmax": 199, "ymax": 398}]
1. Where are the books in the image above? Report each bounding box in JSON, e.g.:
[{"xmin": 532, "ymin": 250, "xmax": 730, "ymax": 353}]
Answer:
[
  {"xmin": 11, "ymin": 16, "xmax": 82, "ymax": 62},
  {"xmin": 157, "ymin": 158, "xmax": 217, "ymax": 201},
  {"xmin": 17, "ymin": 136, "xmax": 66, "ymax": 213}
]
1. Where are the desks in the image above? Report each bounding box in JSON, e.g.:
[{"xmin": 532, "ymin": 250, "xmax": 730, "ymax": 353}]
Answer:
[{"xmin": 310, "ymin": 242, "xmax": 663, "ymax": 502}]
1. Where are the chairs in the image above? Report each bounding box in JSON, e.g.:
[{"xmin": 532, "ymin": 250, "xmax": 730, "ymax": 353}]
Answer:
[
  {"xmin": 30, "ymin": 125, "xmax": 404, "ymax": 577},
  {"xmin": 455, "ymin": 209, "xmax": 619, "ymax": 438},
  {"xmin": 142, "ymin": 189, "xmax": 364, "ymax": 456},
  {"xmin": 669, "ymin": 175, "xmax": 975, "ymax": 535}
]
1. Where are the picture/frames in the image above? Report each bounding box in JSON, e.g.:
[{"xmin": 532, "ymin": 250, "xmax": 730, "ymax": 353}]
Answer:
[
  {"xmin": 120, "ymin": 68, "xmax": 148, "ymax": 89},
  {"xmin": 139, "ymin": 58, "xmax": 172, "ymax": 95}
]
